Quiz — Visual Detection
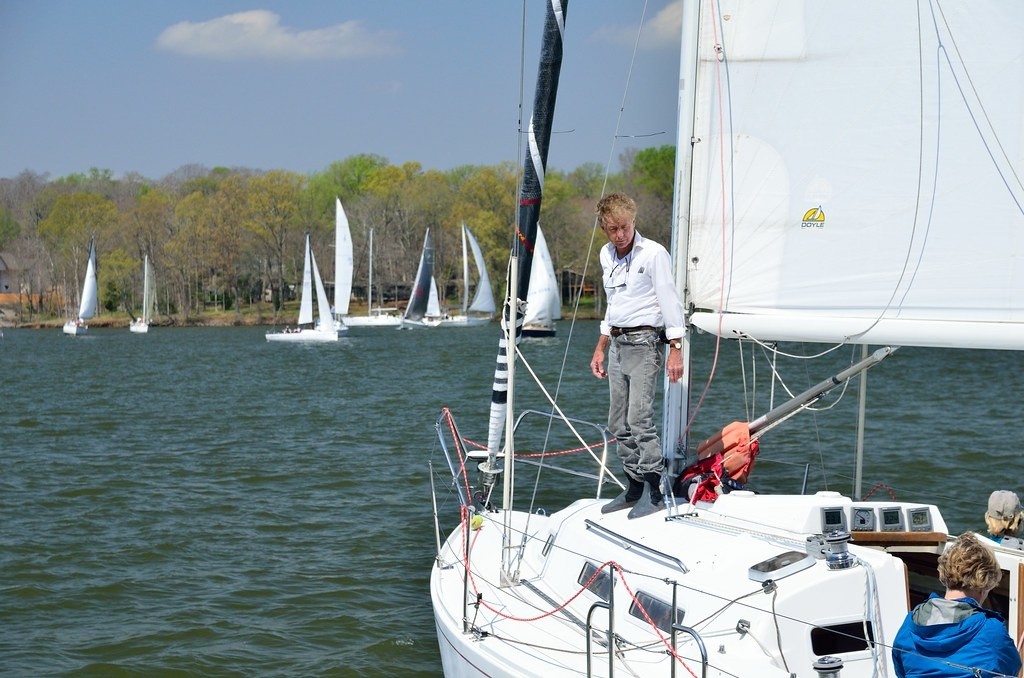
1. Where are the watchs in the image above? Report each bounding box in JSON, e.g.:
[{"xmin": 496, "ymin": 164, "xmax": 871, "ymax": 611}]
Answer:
[{"xmin": 670, "ymin": 343, "xmax": 682, "ymax": 350}]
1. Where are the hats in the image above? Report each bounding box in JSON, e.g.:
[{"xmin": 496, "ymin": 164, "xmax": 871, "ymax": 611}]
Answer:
[{"xmin": 987, "ymin": 490, "xmax": 1024, "ymax": 519}]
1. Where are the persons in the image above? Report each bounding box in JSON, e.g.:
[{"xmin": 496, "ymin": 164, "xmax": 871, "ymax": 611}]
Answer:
[
  {"xmin": 590, "ymin": 192, "xmax": 688, "ymax": 520},
  {"xmin": 985, "ymin": 489, "xmax": 1024, "ymax": 544},
  {"xmin": 891, "ymin": 531, "xmax": 1024, "ymax": 678}
]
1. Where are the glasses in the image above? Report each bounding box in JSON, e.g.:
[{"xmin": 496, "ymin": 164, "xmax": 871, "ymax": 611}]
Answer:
[{"xmin": 604, "ymin": 258, "xmax": 629, "ymax": 290}]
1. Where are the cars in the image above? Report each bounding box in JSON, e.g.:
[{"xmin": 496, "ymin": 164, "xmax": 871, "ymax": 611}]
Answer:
[{"xmin": 554, "ymin": 270, "xmax": 595, "ymax": 299}]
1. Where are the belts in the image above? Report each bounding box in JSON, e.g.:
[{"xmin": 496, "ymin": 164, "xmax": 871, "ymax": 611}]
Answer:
[{"xmin": 611, "ymin": 326, "xmax": 656, "ymax": 336}]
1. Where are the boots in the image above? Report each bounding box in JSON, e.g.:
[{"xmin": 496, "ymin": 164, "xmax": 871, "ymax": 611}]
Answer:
[
  {"xmin": 628, "ymin": 472, "xmax": 666, "ymax": 519},
  {"xmin": 601, "ymin": 472, "xmax": 644, "ymax": 514}
]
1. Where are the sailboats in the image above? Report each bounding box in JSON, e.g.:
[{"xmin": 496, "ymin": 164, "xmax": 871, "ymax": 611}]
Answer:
[
  {"xmin": 398, "ymin": 226, "xmax": 443, "ymax": 330},
  {"xmin": 425, "ymin": 1, "xmax": 1024, "ymax": 678},
  {"xmin": 314, "ymin": 195, "xmax": 354, "ymax": 332},
  {"xmin": 339, "ymin": 227, "xmax": 404, "ymax": 327},
  {"xmin": 63, "ymin": 234, "xmax": 99, "ymax": 335},
  {"xmin": 421, "ymin": 218, "xmax": 496, "ymax": 327},
  {"xmin": 519, "ymin": 220, "xmax": 563, "ymax": 339},
  {"xmin": 129, "ymin": 253, "xmax": 156, "ymax": 334},
  {"xmin": 264, "ymin": 231, "xmax": 339, "ymax": 343}
]
